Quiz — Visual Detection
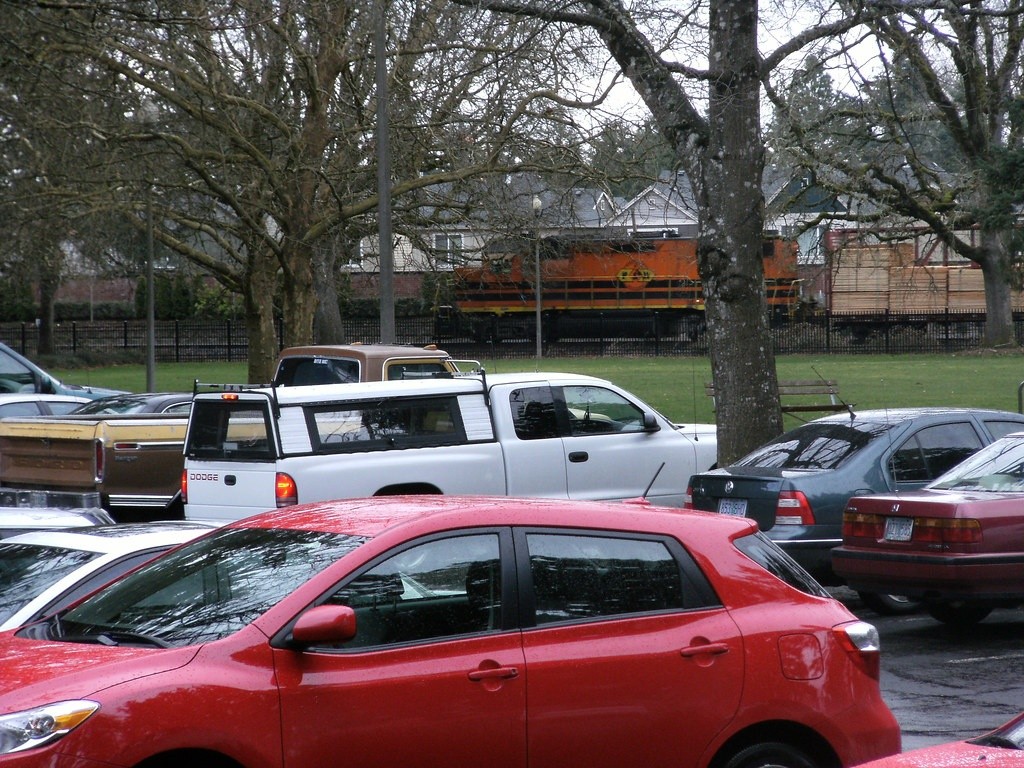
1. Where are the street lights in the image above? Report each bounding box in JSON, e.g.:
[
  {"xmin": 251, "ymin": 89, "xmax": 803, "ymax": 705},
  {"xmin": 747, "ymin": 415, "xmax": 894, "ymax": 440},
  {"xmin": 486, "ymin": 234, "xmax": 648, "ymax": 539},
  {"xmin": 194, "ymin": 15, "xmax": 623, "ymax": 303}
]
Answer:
[
  {"xmin": 138, "ymin": 97, "xmax": 161, "ymax": 393},
  {"xmin": 529, "ymin": 193, "xmax": 544, "ymax": 359}
]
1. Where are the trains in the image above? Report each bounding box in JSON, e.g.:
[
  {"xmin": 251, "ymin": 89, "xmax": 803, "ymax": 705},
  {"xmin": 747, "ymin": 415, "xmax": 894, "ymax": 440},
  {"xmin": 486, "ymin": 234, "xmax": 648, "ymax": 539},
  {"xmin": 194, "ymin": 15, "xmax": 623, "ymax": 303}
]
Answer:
[{"xmin": 453, "ymin": 230, "xmax": 1024, "ymax": 349}]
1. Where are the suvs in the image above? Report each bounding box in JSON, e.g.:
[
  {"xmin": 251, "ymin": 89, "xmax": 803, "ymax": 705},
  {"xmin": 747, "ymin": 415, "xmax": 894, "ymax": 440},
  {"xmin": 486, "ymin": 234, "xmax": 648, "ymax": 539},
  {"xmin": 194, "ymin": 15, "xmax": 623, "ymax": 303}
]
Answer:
[{"xmin": 0, "ymin": 341, "xmax": 134, "ymax": 399}]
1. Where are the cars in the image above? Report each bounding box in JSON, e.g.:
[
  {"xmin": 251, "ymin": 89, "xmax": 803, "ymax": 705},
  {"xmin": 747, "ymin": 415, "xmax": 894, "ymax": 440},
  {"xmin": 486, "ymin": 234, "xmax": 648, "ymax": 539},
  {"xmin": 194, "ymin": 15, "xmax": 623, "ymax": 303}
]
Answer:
[
  {"xmin": 683, "ymin": 405, "xmax": 1024, "ymax": 613},
  {"xmin": 0, "ymin": 490, "xmax": 903, "ymax": 767},
  {"xmin": 833, "ymin": 430, "xmax": 1024, "ymax": 624},
  {"xmin": 0, "ymin": 507, "xmax": 464, "ymax": 630},
  {"xmin": 859, "ymin": 704, "xmax": 1023, "ymax": 767},
  {"xmin": 67, "ymin": 393, "xmax": 196, "ymax": 413},
  {"xmin": 0, "ymin": 393, "xmax": 117, "ymax": 416}
]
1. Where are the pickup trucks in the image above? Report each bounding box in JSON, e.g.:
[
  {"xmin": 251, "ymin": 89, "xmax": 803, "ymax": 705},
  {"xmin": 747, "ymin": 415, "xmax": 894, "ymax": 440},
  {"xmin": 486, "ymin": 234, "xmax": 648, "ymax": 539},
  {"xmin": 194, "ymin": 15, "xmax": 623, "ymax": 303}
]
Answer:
[
  {"xmin": 0, "ymin": 344, "xmax": 610, "ymax": 518},
  {"xmin": 177, "ymin": 372, "xmax": 719, "ymax": 522}
]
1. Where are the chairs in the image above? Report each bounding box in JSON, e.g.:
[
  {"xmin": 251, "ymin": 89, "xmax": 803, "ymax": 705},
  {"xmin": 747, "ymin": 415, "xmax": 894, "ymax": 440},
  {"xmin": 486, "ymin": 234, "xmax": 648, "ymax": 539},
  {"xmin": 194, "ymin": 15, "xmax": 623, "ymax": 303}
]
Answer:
[
  {"xmin": 333, "ymin": 574, "xmax": 416, "ymax": 651},
  {"xmin": 457, "ymin": 554, "xmax": 686, "ymax": 633}
]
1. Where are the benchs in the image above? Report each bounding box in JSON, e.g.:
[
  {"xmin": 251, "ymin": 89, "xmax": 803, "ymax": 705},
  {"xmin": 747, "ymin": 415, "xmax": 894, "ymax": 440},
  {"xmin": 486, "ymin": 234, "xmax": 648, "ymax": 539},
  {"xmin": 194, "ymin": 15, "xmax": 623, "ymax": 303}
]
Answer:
[{"xmin": 703, "ymin": 380, "xmax": 857, "ymax": 413}]
